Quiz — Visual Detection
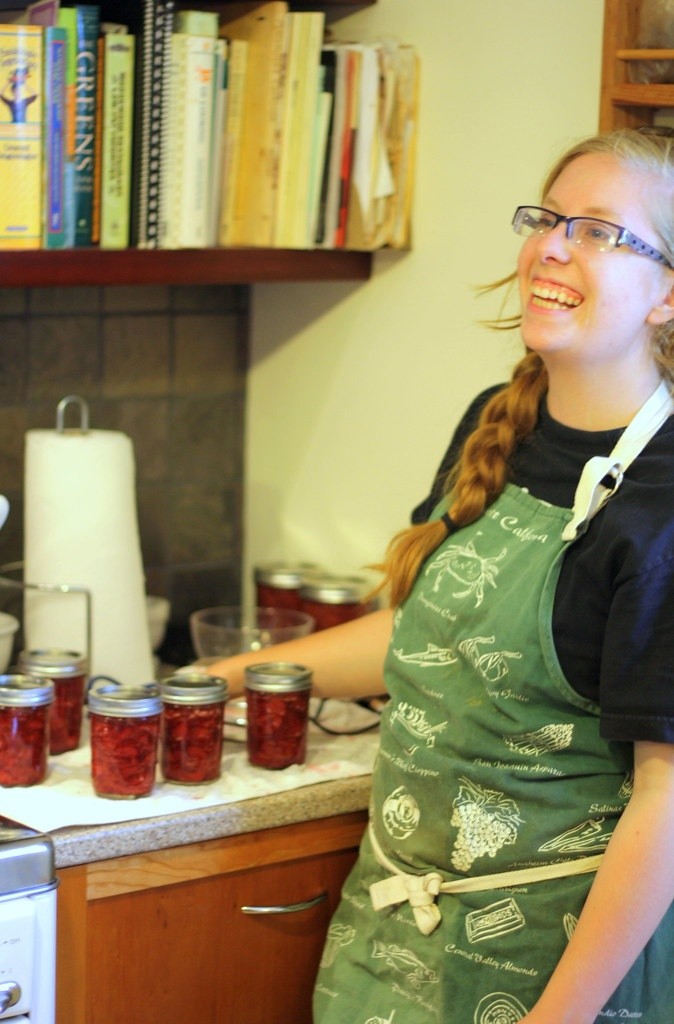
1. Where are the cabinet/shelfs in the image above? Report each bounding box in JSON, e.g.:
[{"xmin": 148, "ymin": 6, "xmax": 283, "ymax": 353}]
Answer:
[{"xmin": 51, "ymin": 810, "xmax": 371, "ymax": 1024}]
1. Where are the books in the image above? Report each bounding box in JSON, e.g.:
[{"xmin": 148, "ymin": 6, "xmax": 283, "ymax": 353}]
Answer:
[{"xmin": 1, "ymin": 0, "xmax": 422, "ymax": 248}]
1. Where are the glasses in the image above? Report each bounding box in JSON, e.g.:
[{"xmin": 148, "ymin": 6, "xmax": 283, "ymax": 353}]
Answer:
[{"xmin": 512, "ymin": 205, "xmax": 674, "ymax": 272}]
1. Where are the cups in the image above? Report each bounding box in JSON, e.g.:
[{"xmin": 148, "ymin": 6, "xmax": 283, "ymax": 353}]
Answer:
[
  {"xmin": 0, "ymin": 674, "xmax": 58, "ymax": 788},
  {"xmin": 86, "ymin": 684, "xmax": 164, "ymax": 801},
  {"xmin": 254, "ymin": 556, "xmax": 379, "ymax": 648},
  {"xmin": 244, "ymin": 661, "xmax": 313, "ymax": 771},
  {"xmin": 18, "ymin": 648, "xmax": 86, "ymax": 754},
  {"xmin": 157, "ymin": 672, "xmax": 228, "ymax": 786}
]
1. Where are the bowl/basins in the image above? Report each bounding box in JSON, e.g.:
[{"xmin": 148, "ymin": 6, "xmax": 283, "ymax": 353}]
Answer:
[
  {"xmin": 0, "ymin": 612, "xmax": 20, "ymax": 675},
  {"xmin": 189, "ymin": 606, "xmax": 317, "ymax": 659}
]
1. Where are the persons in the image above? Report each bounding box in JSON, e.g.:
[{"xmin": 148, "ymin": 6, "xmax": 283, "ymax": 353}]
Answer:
[{"xmin": 209, "ymin": 130, "xmax": 673, "ymax": 1022}]
